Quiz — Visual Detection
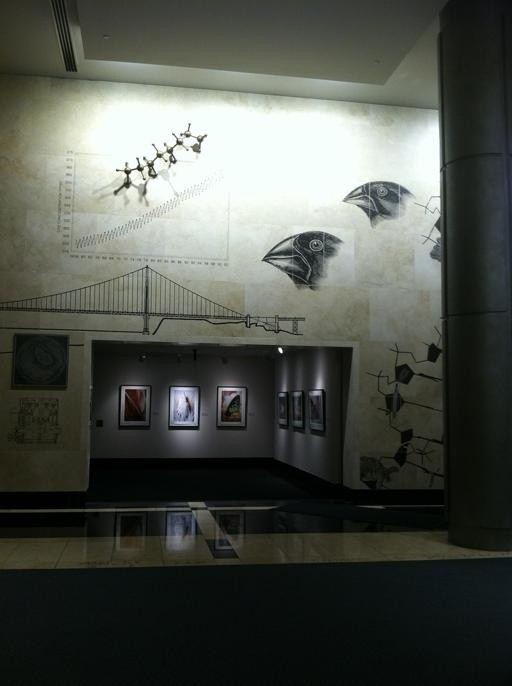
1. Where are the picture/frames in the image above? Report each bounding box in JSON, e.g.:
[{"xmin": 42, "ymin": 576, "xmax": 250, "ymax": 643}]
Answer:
[
  {"xmin": 118, "ymin": 384, "xmax": 248, "ymax": 431},
  {"xmin": 10, "ymin": 333, "xmax": 70, "ymax": 390},
  {"xmin": 277, "ymin": 389, "xmax": 325, "ymax": 432}
]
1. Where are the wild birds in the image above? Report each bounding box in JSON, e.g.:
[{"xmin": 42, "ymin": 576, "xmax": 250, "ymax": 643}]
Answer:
[
  {"xmin": 341, "ymin": 180, "xmax": 413, "ymax": 229},
  {"xmin": 259, "ymin": 226, "xmax": 343, "ymax": 292}
]
